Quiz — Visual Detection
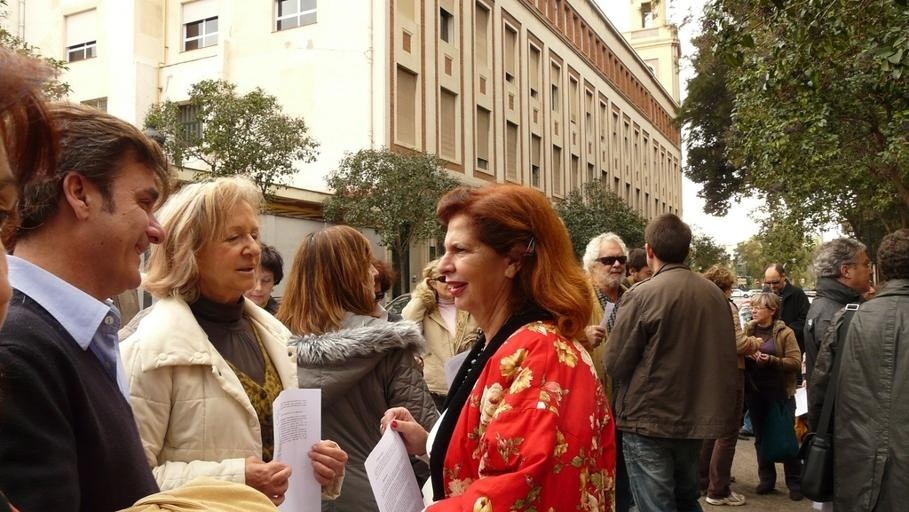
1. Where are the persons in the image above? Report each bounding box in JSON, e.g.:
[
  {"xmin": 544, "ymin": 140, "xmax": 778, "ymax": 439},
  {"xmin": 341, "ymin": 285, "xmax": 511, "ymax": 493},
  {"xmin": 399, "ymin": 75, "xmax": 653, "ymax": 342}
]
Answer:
[
  {"xmin": 371, "ymin": 258, "xmax": 394, "ymax": 319},
  {"xmin": 113, "ymin": 171, "xmax": 349, "ymax": 511},
  {"xmin": 236, "ymin": 241, "xmax": 283, "ymax": 317},
  {"xmin": 401, "ymin": 256, "xmax": 485, "ymax": 415},
  {"xmin": 273, "ymin": 223, "xmax": 442, "ymax": 512},
  {"xmin": 403, "ymin": 213, "xmax": 907, "ymax": 511},
  {"xmin": 378, "ymin": 178, "xmax": 621, "ymax": 512},
  {"xmin": 1, "ymin": 38, "xmax": 67, "ymax": 512},
  {"xmin": 1, "ymin": 103, "xmax": 178, "ymax": 512}
]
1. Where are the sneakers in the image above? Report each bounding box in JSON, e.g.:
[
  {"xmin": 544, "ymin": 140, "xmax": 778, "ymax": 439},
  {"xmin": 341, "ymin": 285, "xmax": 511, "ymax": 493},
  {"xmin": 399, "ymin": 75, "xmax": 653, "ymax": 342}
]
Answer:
[
  {"xmin": 755, "ymin": 483, "xmax": 775, "ymax": 496},
  {"xmin": 705, "ymin": 491, "xmax": 746, "ymax": 506},
  {"xmin": 789, "ymin": 489, "xmax": 804, "ymax": 502}
]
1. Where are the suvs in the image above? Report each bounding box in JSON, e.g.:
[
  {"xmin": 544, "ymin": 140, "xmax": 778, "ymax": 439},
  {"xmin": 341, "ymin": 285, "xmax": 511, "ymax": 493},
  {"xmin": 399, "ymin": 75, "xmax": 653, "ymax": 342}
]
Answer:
[{"xmin": 730, "ymin": 289, "xmax": 764, "ymax": 298}]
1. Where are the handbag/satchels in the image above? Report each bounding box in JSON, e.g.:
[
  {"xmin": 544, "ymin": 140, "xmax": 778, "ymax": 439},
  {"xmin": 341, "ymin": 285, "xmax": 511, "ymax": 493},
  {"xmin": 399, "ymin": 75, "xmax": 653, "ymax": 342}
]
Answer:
[{"xmin": 796, "ymin": 432, "xmax": 834, "ymax": 503}]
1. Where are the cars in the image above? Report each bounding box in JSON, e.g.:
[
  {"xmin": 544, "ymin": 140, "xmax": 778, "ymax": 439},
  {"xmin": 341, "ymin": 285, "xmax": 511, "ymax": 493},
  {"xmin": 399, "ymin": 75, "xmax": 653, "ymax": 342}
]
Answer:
[{"xmin": 804, "ymin": 290, "xmax": 819, "ymax": 304}]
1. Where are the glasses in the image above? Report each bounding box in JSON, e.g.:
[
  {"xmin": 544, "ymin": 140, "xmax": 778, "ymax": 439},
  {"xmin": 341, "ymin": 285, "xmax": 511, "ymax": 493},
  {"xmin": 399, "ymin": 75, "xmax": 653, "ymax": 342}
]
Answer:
[
  {"xmin": 592, "ymin": 256, "xmax": 627, "ymax": 265},
  {"xmin": 430, "ymin": 275, "xmax": 446, "ymax": 283},
  {"xmin": 764, "ymin": 277, "xmax": 782, "ymax": 284}
]
